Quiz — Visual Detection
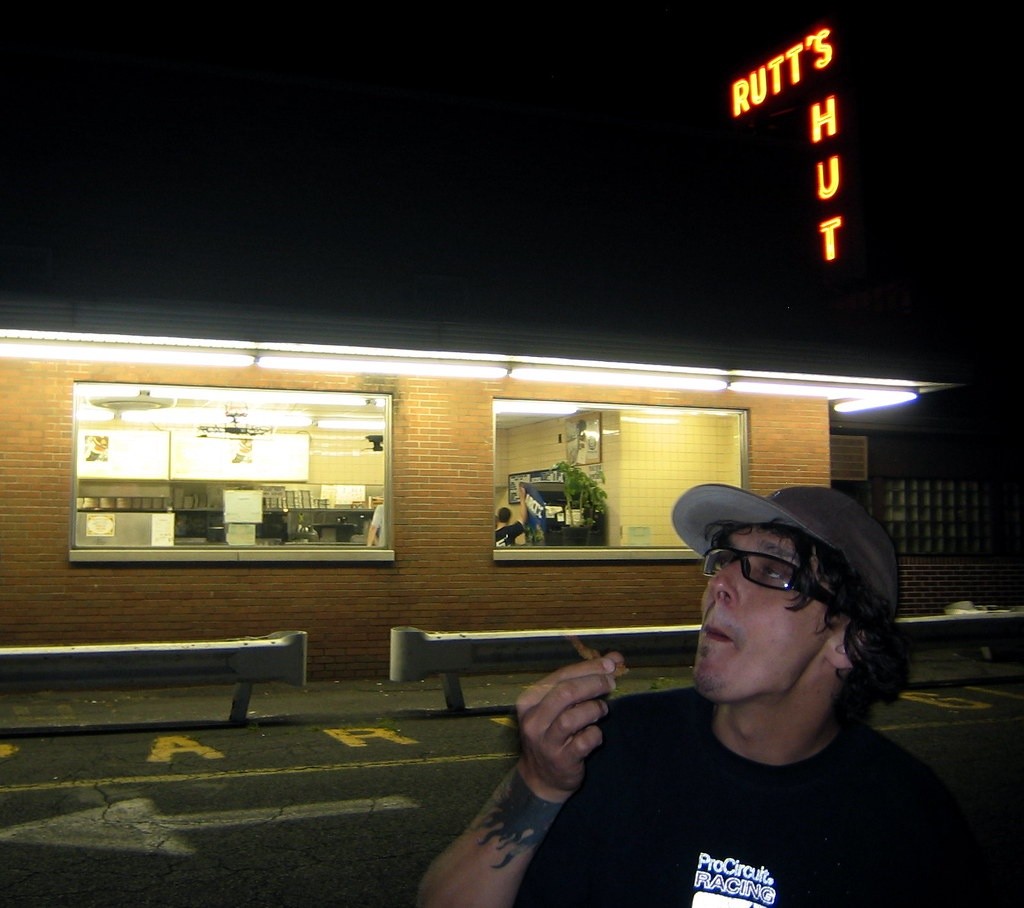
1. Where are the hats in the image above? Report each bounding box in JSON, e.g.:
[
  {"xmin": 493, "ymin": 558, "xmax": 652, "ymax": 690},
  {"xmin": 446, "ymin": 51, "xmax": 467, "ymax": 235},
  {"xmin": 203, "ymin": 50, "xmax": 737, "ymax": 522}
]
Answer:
[{"xmin": 672, "ymin": 482, "xmax": 899, "ymax": 623}]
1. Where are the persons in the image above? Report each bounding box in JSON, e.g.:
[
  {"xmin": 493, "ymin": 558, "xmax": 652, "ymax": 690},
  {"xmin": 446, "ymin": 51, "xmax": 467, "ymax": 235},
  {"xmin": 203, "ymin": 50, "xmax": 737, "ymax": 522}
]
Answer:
[
  {"xmin": 415, "ymin": 486, "xmax": 1016, "ymax": 907},
  {"xmin": 496, "ymin": 480, "xmax": 530, "ymax": 547},
  {"xmin": 367, "ymin": 499, "xmax": 385, "ymax": 549}
]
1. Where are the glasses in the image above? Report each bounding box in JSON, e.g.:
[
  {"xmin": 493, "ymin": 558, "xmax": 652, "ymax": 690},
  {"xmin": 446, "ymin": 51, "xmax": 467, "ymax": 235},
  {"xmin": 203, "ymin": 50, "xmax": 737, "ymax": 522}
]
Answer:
[{"xmin": 701, "ymin": 547, "xmax": 838, "ymax": 610}]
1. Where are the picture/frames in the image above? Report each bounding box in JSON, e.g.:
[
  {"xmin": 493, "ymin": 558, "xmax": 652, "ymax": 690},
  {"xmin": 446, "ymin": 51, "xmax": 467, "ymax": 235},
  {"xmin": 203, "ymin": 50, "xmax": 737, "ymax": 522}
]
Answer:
[
  {"xmin": 508, "ymin": 469, "xmax": 566, "ymax": 504},
  {"xmin": 565, "ymin": 412, "xmax": 602, "ymax": 466}
]
